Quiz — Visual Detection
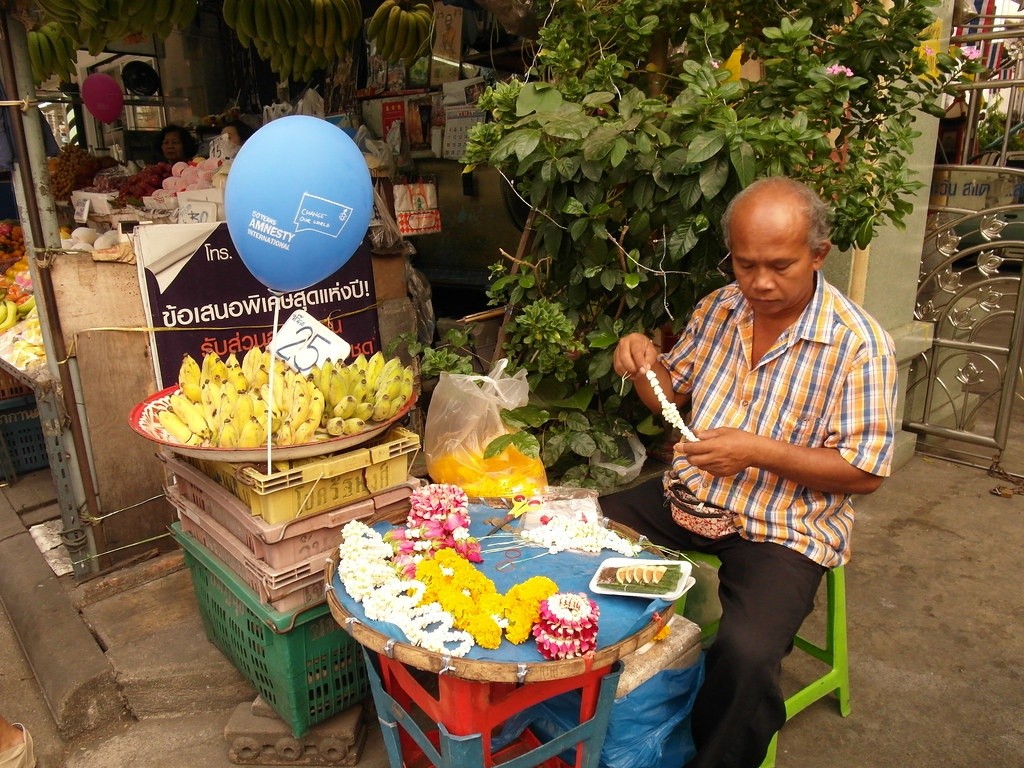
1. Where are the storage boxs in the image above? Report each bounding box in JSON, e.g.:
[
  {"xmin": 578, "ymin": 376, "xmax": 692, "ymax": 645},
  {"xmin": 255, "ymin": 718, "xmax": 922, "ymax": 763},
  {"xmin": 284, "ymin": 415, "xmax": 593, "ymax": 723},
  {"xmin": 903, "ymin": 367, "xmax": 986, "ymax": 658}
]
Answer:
[
  {"xmin": 142, "ymin": 196, "xmax": 178, "ymax": 209},
  {"xmin": 0, "ymin": 368, "xmax": 52, "ymax": 488},
  {"xmin": 155, "ymin": 425, "xmax": 429, "ymax": 737},
  {"xmin": 177, "ymin": 188, "xmax": 225, "ymax": 220},
  {"xmin": 70, "ymin": 187, "xmax": 119, "ymax": 215},
  {"xmin": 603, "ymin": 613, "xmax": 703, "ymax": 760}
]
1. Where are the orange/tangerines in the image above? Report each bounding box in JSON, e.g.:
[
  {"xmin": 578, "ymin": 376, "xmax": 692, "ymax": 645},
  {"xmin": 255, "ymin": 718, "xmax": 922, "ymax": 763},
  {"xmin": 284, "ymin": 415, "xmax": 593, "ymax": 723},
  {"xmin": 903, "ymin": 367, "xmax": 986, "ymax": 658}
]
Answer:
[{"xmin": 0, "ymin": 256, "xmax": 29, "ymax": 289}]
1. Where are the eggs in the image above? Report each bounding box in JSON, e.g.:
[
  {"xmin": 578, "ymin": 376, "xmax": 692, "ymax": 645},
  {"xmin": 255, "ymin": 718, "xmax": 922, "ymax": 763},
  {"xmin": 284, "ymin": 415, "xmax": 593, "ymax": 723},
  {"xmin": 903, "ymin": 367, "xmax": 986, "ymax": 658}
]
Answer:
[{"xmin": 616, "ymin": 565, "xmax": 667, "ymax": 585}]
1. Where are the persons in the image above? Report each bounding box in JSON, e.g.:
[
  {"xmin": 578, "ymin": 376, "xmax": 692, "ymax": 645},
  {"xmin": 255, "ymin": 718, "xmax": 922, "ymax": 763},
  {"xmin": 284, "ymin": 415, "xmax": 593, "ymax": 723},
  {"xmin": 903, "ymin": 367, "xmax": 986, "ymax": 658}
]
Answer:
[
  {"xmin": 198, "ymin": 119, "xmax": 255, "ymax": 161},
  {"xmin": 596, "ymin": 176, "xmax": 897, "ymax": 768},
  {"xmin": 147, "ymin": 124, "xmax": 198, "ymax": 164}
]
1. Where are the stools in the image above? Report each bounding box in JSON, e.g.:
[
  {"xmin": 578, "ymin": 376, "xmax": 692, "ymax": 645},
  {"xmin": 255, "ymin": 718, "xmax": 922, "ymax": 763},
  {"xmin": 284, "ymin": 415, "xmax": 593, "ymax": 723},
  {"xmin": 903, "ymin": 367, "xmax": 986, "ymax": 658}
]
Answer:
[
  {"xmin": 361, "ymin": 646, "xmax": 625, "ymax": 768},
  {"xmin": 676, "ymin": 550, "xmax": 852, "ymax": 768}
]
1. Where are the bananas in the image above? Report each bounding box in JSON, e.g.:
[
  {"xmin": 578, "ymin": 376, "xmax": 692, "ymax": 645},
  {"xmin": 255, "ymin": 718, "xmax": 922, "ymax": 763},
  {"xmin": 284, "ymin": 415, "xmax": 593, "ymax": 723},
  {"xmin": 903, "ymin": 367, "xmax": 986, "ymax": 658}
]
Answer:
[
  {"xmin": 26, "ymin": 1, "xmax": 201, "ymax": 90},
  {"xmin": 0, "ymin": 294, "xmax": 37, "ymax": 332},
  {"xmin": 223, "ymin": 0, "xmax": 435, "ymax": 83},
  {"xmin": 157, "ymin": 344, "xmax": 413, "ymax": 448}
]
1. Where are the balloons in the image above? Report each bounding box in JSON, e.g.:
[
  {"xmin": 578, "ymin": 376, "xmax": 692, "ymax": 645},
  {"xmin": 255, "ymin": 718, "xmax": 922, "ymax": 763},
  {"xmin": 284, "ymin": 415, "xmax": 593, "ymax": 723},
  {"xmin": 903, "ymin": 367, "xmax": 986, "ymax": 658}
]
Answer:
[
  {"xmin": 224, "ymin": 115, "xmax": 373, "ymax": 292},
  {"xmin": 82, "ymin": 73, "xmax": 124, "ymax": 124}
]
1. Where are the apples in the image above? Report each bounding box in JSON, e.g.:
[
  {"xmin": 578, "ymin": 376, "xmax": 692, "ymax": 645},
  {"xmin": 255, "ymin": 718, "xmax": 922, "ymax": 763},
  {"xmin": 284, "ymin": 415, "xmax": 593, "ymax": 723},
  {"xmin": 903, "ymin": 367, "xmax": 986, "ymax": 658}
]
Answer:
[{"xmin": 153, "ymin": 157, "xmax": 223, "ymax": 196}]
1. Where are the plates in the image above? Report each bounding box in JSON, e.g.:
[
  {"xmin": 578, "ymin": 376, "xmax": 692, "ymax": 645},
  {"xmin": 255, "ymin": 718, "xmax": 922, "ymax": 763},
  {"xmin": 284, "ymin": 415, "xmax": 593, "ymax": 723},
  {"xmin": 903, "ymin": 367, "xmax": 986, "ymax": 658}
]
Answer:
[
  {"xmin": 128, "ymin": 377, "xmax": 417, "ymax": 462},
  {"xmin": 589, "ymin": 558, "xmax": 696, "ymax": 601}
]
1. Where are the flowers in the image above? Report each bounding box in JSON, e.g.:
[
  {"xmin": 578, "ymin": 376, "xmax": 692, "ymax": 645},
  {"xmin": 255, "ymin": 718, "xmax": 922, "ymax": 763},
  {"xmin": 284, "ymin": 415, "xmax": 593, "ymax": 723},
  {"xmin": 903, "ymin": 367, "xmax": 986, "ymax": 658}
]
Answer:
[
  {"xmin": 646, "ymin": 368, "xmax": 701, "ymax": 444},
  {"xmin": 339, "ymin": 484, "xmax": 641, "ymax": 659}
]
